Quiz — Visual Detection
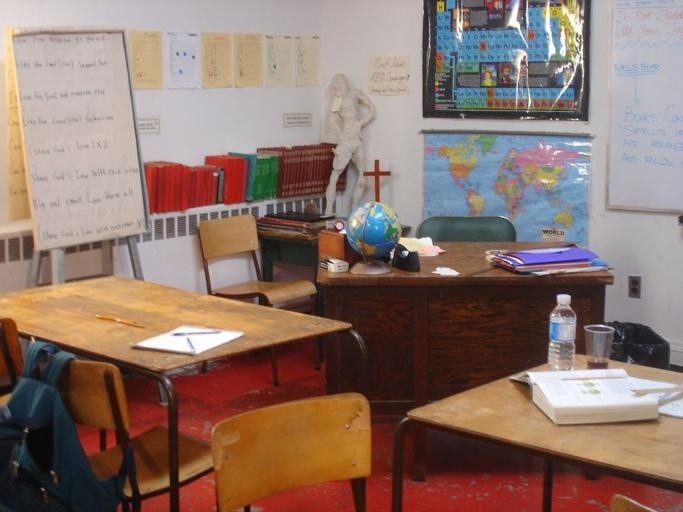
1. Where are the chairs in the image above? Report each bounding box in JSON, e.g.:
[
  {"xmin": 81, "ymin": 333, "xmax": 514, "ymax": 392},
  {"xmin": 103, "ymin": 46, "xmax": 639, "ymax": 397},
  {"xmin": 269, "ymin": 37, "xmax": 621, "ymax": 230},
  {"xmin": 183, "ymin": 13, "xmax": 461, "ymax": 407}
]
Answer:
[
  {"xmin": 414, "ymin": 216, "xmax": 516, "ymax": 242},
  {"xmin": 0, "ymin": 317, "xmax": 24, "ymax": 398},
  {"xmin": 198, "ymin": 214, "xmax": 322, "ymax": 386},
  {"xmin": 611, "ymin": 493, "xmax": 657, "ymax": 512},
  {"xmin": 210, "ymin": 392, "xmax": 373, "ymax": 511},
  {"xmin": 27, "ymin": 335, "xmax": 214, "ymax": 512}
]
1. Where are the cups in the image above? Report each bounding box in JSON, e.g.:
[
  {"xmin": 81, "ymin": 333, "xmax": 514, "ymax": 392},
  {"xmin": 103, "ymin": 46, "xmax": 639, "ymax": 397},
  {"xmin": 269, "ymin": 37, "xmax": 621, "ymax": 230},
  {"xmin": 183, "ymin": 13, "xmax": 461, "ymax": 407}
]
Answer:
[{"xmin": 584, "ymin": 324, "xmax": 615, "ymax": 369}]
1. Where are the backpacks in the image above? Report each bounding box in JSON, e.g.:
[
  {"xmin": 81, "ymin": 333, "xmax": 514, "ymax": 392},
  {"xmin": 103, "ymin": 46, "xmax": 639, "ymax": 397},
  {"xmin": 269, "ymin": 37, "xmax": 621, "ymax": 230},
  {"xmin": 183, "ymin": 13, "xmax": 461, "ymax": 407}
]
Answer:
[{"xmin": 0, "ymin": 340, "xmax": 132, "ymax": 511}]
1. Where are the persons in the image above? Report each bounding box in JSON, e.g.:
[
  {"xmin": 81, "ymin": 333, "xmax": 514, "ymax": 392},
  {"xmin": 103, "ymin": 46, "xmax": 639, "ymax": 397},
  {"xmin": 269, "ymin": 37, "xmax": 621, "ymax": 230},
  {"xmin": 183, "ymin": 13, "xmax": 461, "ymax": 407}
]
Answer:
[{"xmin": 325, "ymin": 73, "xmax": 376, "ymax": 216}]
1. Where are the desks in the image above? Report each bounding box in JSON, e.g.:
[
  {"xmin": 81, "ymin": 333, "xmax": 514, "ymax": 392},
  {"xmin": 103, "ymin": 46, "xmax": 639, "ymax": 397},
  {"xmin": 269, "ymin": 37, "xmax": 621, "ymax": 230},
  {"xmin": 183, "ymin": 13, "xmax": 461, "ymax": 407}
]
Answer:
[
  {"xmin": 315, "ymin": 241, "xmax": 614, "ymax": 482},
  {"xmin": 258, "ymin": 224, "xmax": 411, "ymax": 360},
  {"xmin": 392, "ymin": 353, "xmax": 683, "ymax": 512},
  {"xmin": 0, "ymin": 276, "xmax": 368, "ymax": 511}
]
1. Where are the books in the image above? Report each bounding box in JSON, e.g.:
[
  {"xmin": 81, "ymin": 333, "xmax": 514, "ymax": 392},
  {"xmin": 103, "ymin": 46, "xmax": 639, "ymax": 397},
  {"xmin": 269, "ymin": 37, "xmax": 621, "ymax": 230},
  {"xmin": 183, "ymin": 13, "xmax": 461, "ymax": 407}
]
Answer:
[
  {"xmin": 508, "ymin": 368, "xmax": 660, "ymax": 425},
  {"xmin": 142, "ymin": 142, "xmax": 347, "ymax": 241}
]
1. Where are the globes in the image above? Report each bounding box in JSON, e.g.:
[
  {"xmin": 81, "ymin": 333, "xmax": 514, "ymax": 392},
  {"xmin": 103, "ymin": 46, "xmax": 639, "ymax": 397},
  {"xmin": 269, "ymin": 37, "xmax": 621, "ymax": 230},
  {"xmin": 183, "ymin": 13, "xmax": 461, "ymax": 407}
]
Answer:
[{"xmin": 345, "ymin": 201, "xmax": 403, "ymax": 275}]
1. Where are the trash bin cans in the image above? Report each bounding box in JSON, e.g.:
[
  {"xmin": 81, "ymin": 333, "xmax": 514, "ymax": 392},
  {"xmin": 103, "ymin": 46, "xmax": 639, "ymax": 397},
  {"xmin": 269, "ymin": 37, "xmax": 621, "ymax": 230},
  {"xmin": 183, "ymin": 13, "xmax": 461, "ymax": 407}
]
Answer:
[{"xmin": 605, "ymin": 323, "xmax": 671, "ymax": 370}]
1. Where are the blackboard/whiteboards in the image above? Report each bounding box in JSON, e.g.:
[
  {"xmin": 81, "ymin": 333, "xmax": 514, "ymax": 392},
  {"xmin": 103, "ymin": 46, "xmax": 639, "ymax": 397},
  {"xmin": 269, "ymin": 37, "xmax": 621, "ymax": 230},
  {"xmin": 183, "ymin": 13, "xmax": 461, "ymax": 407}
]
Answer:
[
  {"xmin": 605, "ymin": 0, "xmax": 683, "ymax": 213},
  {"xmin": 11, "ymin": 28, "xmax": 152, "ymax": 250}
]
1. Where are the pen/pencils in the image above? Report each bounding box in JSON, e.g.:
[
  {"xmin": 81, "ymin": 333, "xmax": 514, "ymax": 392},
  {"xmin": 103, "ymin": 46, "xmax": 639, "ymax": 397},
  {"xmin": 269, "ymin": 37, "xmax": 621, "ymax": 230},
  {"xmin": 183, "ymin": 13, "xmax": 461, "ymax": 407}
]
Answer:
[
  {"xmin": 115, "ymin": 319, "xmax": 146, "ymax": 329},
  {"xmin": 187, "ymin": 337, "xmax": 195, "ymax": 350},
  {"xmin": 95, "ymin": 314, "xmax": 137, "ymax": 323},
  {"xmin": 173, "ymin": 330, "xmax": 222, "ymax": 335}
]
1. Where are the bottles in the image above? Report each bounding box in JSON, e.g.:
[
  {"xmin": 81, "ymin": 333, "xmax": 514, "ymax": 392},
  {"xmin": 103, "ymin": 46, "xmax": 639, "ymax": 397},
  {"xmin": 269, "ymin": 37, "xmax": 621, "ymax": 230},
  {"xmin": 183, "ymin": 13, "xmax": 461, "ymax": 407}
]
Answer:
[{"xmin": 547, "ymin": 294, "xmax": 577, "ymax": 371}]
